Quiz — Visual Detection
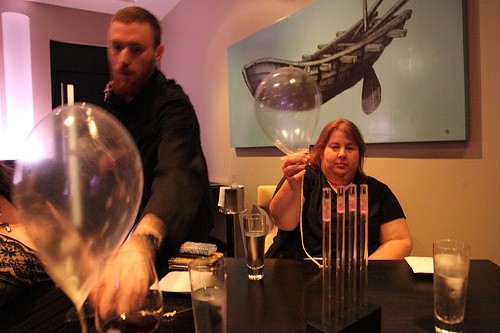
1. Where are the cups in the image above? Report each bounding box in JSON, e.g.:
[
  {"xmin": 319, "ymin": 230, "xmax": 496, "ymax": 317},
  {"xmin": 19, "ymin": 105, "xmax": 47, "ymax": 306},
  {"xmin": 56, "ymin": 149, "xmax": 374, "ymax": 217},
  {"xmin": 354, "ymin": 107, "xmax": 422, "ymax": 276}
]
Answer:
[
  {"xmin": 433, "ymin": 240, "xmax": 469, "ymax": 333},
  {"xmin": 188, "ymin": 256, "xmax": 227, "ymax": 333},
  {"xmin": 243, "ymin": 214, "xmax": 266, "ymax": 280},
  {"xmin": 94, "ymin": 251, "xmax": 163, "ymax": 333}
]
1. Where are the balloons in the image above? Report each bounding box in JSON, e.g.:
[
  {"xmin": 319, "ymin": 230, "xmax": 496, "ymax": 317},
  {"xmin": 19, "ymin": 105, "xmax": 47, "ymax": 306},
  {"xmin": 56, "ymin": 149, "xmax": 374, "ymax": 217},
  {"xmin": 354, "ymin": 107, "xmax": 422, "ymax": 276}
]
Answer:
[
  {"xmin": 13, "ymin": 105, "xmax": 144, "ymax": 312},
  {"xmin": 253, "ymin": 67, "xmax": 321, "ymax": 160}
]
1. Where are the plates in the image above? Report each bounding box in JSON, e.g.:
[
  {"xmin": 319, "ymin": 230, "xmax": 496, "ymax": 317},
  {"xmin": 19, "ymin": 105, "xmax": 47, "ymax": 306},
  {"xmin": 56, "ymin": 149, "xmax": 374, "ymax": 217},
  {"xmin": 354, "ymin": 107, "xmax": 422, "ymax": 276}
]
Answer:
[
  {"xmin": 404, "ymin": 257, "xmax": 434, "ymax": 273},
  {"xmin": 150, "ymin": 271, "xmax": 227, "ymax": 292}
]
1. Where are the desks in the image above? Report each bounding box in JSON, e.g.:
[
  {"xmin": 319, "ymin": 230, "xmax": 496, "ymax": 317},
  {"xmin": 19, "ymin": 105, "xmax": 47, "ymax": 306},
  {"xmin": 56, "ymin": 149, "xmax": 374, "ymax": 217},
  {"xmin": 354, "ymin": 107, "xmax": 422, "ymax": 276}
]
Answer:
[{"xmin": 162, "ymin": 257, "xmax": 500, "ymax": 333}]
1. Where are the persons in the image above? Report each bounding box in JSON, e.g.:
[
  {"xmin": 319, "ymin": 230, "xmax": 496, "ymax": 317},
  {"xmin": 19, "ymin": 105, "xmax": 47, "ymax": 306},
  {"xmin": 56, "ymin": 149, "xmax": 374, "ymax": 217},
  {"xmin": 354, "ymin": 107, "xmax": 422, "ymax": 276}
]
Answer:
[
  {"xmin": 68, "ymin": 5, "xmax": 213, "ymax": 324},
  {"xmin": 262, "ymin": 119, "xmax": 413, "ymax": 259},
  {"xmin": 0, "ymin": 149, "xmax": 106, "ymax": 333}
]
